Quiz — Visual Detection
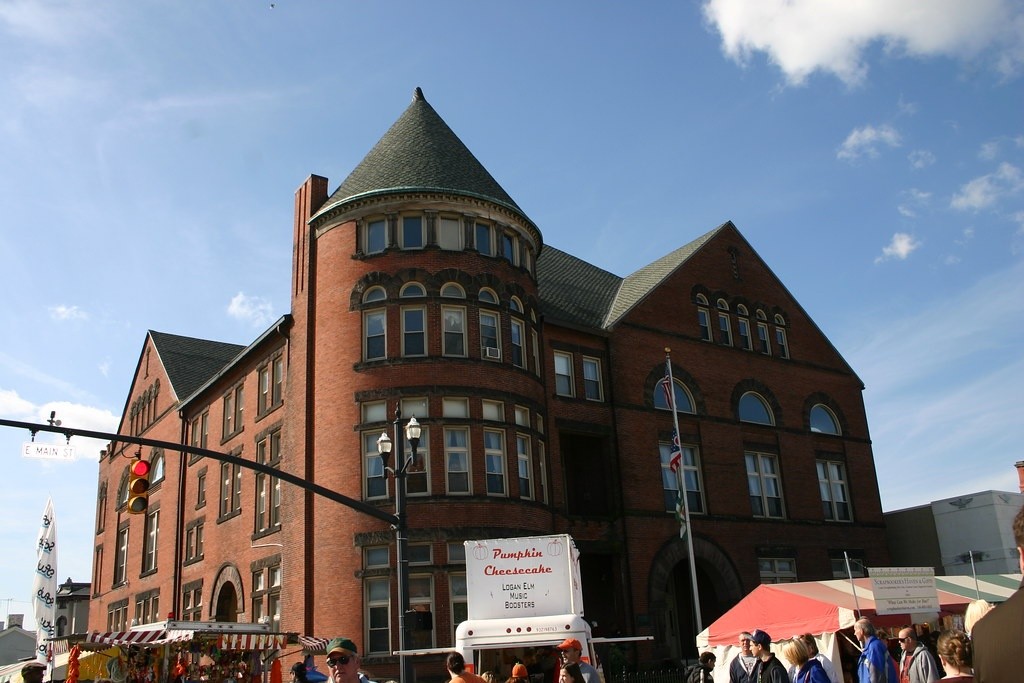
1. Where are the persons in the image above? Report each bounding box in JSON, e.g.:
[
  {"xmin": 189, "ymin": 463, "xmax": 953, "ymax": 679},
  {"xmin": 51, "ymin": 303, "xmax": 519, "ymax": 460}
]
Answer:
[
  {"xmin": 854, "ymin": 616, "xmax": 940, "ymax": 683},
  {"xmin": 929, "ymin": 597, "xmax": 991, "ymax": 683},
  {"xmin": 728, "ymin": 629, "xmax": 838, "ymax": 683},
  {"xmin": 972, "ymin": 504, "xmax": 1024, "ymax": 683},
  {"xmin": 687, "ymin": 651, "xmax": 716, "ymax": 683},
  {"xmin": 290, "ymin": 637, "xmax": 397, "ymax": 683},
  {"xmin": 556, "ymin": 637, "xmax": 601, "ymax": 683},
  {"xmin": 506, "ymin": 662, "xmax": 528, "ymax": 683},
  {"xmin": 441, "ymin": 651, "xmax": 498, "ymax": 683},
  {"xmin": 21, "ymin": 663, "xmax": 47, "ymax": 683}
]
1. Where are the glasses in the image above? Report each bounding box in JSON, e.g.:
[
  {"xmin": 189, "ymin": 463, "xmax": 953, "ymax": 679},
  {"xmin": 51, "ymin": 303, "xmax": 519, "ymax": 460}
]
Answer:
[
  {"xmin": 559, "ymin": 649, "xmax": 570, "ymax": 653},
  {"xmin": 896, "ymin": 636, "xmax": 912, "ymax": 642},
  {"xmin": 326, "ymin": 655, "xmax": 353, "ymax": 667},
  {"xmin": 881, "ymin": 637, "xmax": 889, "ymax": 642},
  {"xmin": 290, "ymin": 672, "xmax": 293, "ymax": 674}
]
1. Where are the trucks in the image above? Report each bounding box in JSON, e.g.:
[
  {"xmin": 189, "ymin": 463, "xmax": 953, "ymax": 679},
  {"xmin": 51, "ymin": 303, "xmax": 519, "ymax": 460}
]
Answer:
[{"xmin": 451, "ymin": 534, "xmax": 603, "ymax": 683}]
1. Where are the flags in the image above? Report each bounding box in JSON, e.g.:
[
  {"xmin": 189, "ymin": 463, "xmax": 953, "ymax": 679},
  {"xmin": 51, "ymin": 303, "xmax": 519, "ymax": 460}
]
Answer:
[
  {"xmin": 669, "ymin": 418, "xmax": 682, "ymax": 472},
  {"xmin": 675, "ymin": 467, "xmax": 687, "ymax": 538},
  {"xmin": 662, "ymin": 354, "xmax": 674, "ymax": 410}
]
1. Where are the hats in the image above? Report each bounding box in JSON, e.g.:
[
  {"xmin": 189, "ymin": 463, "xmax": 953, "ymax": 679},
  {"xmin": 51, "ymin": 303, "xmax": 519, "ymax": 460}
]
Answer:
[
  {"xmin": 744, "ymin": 628, "xmax": 772, "ymax": 646},
  {"xmin": 556, "ymin": 638, "xmax": 583, "ymax": 651},
  {"xmin": 511, "ymin": 661, "xmax": 528, "ymax": 678},
  {"xmin": 21, "ymin": 662, "xmax": 47, "ymax": 677},
  {"xmin": 325, "ymin": 637, "xmax": 358, "ymax": 658}
]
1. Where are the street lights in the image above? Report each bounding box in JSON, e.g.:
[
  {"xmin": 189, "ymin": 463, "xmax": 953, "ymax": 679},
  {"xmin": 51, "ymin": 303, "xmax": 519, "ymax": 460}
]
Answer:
[{"xmin": 375, "ymin": 399, "xmax": 430, "ymax": 681}]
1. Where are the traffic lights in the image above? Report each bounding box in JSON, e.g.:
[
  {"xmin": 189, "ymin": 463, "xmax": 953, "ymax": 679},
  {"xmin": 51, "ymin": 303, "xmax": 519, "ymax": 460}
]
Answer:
[{"xmin": 127, "ymin": 459, "xmax": 153, "ymax": 515}]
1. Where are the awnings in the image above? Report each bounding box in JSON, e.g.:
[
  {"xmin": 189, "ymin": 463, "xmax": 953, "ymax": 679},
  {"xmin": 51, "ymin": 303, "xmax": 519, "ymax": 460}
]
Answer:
[{"xmin": 43, "ymin": 629, "xmax": 332, "ymax": 663}]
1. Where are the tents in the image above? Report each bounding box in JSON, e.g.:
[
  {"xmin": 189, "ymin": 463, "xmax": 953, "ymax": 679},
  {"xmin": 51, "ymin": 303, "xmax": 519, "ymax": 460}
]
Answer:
[{"xmin": 694, "ymin": 574, "xmax": 1024, "ymax": 683}]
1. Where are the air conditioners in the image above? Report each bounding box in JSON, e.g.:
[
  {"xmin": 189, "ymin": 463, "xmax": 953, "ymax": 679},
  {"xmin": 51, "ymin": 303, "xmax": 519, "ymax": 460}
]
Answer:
[{"xmin": 484, "ymin": 347, "xmax": 500, "ymax": 359}]
